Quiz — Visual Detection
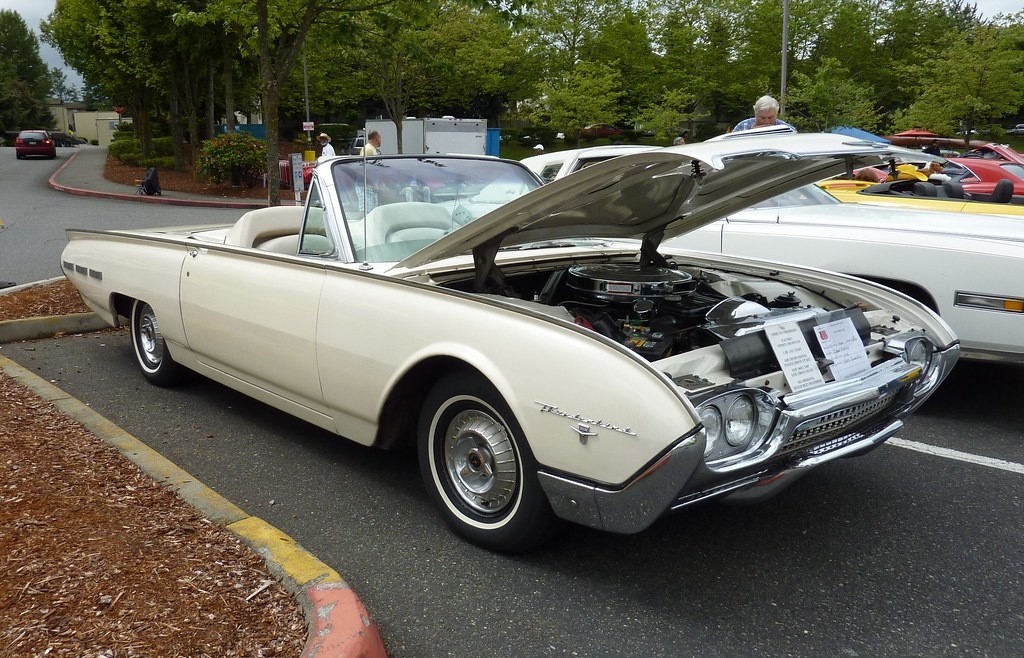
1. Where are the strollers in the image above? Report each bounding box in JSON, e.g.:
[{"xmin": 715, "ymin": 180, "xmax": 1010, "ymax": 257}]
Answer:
[{"xmin": 134, "ymin": 167, "xmax": 161, "ymax": 196}]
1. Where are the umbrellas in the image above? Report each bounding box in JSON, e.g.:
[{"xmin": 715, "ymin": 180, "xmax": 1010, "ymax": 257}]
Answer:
[{"xmin": 893, "ymin": 128, "xmax": 938, "ymax": 150}]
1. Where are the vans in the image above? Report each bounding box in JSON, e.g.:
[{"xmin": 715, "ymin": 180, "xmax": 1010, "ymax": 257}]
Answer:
[{"xmin": 1011, "ymin": 123, "xmax": 1024, "ymax": 135}]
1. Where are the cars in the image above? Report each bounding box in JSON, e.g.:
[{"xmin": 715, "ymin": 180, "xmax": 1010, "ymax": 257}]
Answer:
[
  {"xmin": 340, "ymin": 137, "xmax": 366, "ymax": 154},
  {"xmin": 436, "ymin": 124, "xmax": 1024, "ymax": 367},
  {"xmin": 965, "ymin": 128, "xmax": 991, "ymax": 136},
  {"xmin": 16, "ymin": 129, "xmax": 57, "ymax": 160},
  {"xmin": 61, "ymin": 133, "xmax": 961, "ymax": 562},
  {"xmin": 581, "ymin": 123, "xmax": 626, "ymax": 142}
]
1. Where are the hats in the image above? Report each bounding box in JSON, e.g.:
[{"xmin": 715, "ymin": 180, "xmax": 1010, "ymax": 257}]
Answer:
[
  {"xmin": 316, "ymin": 133, "xmax": 331, "ymax": 142},
  {"xmin": 884, "ymin": 165, "xmax": 928, "ymax": 182},
  {"xmin": 533, "ymin": 144, "xmax": 544, "ymax": 150}
]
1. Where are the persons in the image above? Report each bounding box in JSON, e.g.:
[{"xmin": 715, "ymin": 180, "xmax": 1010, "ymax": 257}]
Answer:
[
  {"xmin": 673, "ymin": 136, "xmax": 685, "ymax": 146},
  {"xmin": 395, "ymin": 161, "xmax": 431, "ymax": 203},
  {"xmin": 533, "ymin": 144, "xmax": 551, "ymax": 178},
  {"xmin": 314, "ymin": 133, "xmax": 350, "ymax": 206},
  {"xmin": 730, "ymin": 95, "xmax": 797, "ymax": 135},
  {"xmin": 922, "ymin": 139, "xmax": 940, "ymax": 156},
  {"xmin": 355, "ymin": 128, "xmax": 382, "ymax": 219}
]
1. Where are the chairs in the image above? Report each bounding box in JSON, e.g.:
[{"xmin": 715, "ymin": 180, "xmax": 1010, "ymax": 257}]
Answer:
[
  {"xmin": 361, "ymin": 201, "xmax": 454, "ymax": 248},
  {"xmin": 224, "ymin": 206, "xmax": 329, "ymax": 255}
]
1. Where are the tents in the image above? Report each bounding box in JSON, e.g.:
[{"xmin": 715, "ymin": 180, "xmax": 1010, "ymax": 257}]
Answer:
[{"xmin": 820, "ymin": 124, "xmax": 891, "ymax": 145}]
